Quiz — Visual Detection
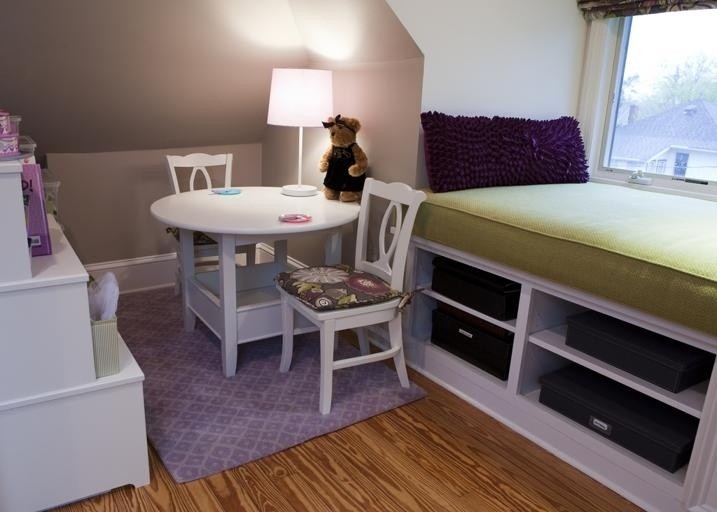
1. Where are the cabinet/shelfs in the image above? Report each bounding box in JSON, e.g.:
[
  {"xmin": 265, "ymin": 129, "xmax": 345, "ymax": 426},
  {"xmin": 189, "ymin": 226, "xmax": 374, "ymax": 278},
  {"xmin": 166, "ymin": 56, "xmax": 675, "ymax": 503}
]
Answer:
[{"xmin": 0, "ymin": 160, "xmax": 152, "ymax": 512}]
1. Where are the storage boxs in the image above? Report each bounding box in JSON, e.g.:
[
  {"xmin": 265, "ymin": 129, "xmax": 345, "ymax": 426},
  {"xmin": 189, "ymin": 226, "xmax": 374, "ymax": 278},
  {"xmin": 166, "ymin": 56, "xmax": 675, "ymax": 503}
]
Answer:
[
  {"xmin": 432, "ymin": 256, "xmax": 521, "ymax": 321},
  {"xmin": 432, "ymin": 308, "xmax": 514, "ymax": 380}
]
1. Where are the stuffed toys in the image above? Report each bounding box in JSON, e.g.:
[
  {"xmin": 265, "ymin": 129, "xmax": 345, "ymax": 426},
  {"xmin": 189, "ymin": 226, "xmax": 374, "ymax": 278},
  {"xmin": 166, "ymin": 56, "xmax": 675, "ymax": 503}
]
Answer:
[{"xmin": 318, "ymin": 113, "xmax": 369, "ymax": 204}]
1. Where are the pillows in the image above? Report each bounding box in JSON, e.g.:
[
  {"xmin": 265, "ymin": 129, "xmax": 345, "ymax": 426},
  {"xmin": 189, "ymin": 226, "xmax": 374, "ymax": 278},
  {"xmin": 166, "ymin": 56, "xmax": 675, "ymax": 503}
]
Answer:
[{"xmin": 420, "ymin": 110, "xmax": 590, "ymax": 193}]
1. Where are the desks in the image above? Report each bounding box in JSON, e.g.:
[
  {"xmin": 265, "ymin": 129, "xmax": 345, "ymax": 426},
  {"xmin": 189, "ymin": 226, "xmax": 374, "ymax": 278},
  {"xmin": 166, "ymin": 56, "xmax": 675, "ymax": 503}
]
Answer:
[{"xmin": 150, "ymin": 186, "xmax": 360, "ymax": 378}]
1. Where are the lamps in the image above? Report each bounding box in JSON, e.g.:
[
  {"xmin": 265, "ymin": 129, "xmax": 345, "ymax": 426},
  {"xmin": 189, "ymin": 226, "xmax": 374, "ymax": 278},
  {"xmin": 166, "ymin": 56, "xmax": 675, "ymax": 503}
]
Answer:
[{"xmin": 267, "ymin": 68, "xmax": 334, "ymax": 197}]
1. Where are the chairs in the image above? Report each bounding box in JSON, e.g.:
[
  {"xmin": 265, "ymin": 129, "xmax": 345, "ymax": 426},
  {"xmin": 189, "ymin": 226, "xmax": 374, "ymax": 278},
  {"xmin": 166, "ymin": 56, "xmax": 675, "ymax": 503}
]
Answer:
[
  {"xmin": 272, "ymin": 177, "xmax": 427, "ymax": 416},
  {"xmin": 166, "ymin": 152, "xmax": 256, "ymax": 297}
]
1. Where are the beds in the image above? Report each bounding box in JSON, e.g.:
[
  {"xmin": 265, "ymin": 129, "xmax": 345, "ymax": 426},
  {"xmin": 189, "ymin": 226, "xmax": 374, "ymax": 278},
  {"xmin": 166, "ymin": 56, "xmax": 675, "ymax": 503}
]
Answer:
[{"xmin": 402, "ymin": 181, "xmax": 717, "ymax": 512}]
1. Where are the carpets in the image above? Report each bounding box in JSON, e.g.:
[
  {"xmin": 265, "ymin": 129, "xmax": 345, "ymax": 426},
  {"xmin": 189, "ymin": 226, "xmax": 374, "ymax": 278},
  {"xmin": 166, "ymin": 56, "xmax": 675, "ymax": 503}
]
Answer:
[{"xmin": 116, "ymin": 286, "xmax": 429, "ymax": 484}]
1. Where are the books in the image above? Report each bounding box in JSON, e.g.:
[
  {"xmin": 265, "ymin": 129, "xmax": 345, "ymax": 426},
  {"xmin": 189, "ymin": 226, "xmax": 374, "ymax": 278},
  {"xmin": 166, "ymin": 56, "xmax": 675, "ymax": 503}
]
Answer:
[{"xmin": 20, "ymin": 163, "xmax": 54, "ymax": 258}]
